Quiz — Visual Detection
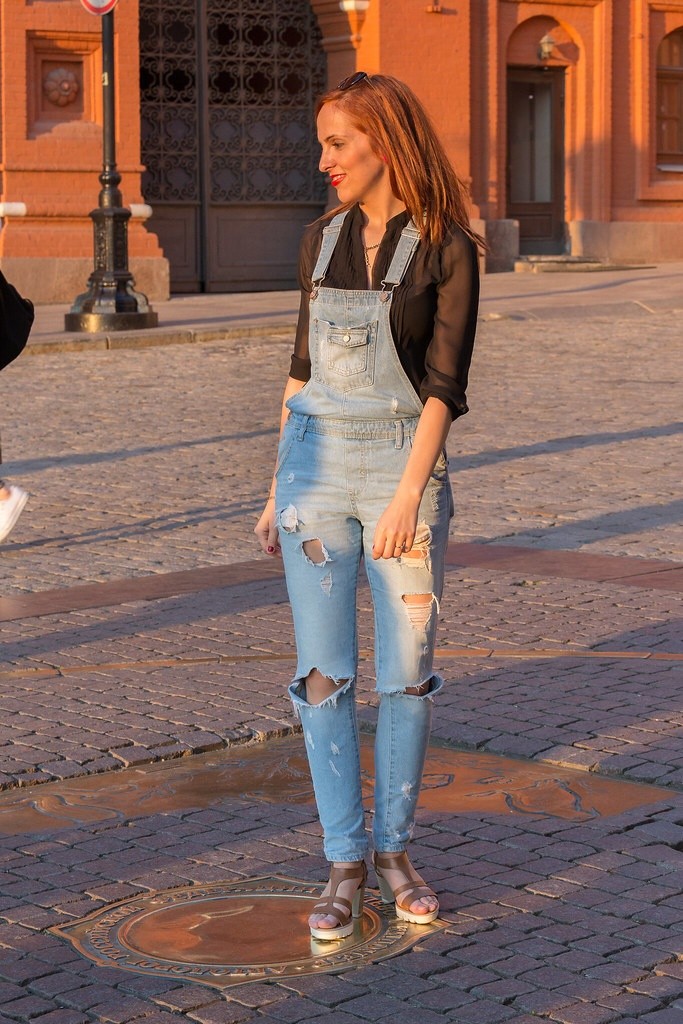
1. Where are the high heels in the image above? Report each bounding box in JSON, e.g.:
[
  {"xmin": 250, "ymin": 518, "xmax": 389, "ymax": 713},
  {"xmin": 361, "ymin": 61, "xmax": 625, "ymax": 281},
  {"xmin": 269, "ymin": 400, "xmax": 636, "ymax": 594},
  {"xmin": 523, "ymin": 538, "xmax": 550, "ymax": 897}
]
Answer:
[
  {"xmin": 371, "ymin": 848, "xmax": 439, "ymax": 925},
  {"xmin": 309, "ymin": 859, "xmax": 368, "ymax": 940}
]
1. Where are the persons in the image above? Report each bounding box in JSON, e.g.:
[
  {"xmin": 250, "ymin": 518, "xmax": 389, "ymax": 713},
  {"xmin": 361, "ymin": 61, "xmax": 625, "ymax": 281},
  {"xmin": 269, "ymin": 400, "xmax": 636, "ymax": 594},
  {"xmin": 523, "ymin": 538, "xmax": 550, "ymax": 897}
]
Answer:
[
  {"xmin": 254, "ymin": 71, "xmax": 481, "ymax": 939},
  {"xmin": 0, "ymin": 270, "xmax": 33, "ymax": 543}
]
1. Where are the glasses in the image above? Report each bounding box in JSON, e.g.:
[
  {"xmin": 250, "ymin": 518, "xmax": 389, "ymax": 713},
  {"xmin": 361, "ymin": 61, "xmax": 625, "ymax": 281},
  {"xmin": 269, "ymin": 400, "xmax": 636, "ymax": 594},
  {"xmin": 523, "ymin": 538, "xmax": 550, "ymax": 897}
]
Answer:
[{"xmin": 337, "ymin": 71, "xmax": 380, "ymax": 96}]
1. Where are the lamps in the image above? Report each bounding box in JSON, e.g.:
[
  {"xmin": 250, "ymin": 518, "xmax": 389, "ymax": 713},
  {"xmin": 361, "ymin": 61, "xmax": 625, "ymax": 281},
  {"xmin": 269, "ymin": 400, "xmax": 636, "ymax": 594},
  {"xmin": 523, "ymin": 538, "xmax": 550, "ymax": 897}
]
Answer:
[{"xmin": 539, "ymin": 34, "xmax": 555, "ymax": 59}]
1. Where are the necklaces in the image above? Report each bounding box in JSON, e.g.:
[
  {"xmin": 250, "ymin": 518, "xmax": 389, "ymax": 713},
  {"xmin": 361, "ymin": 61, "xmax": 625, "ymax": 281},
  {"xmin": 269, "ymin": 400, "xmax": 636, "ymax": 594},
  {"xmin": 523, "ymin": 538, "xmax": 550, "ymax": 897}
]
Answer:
[{"xmin": 364, "ymin": 245, "xmax": 379, "ymax": 266}]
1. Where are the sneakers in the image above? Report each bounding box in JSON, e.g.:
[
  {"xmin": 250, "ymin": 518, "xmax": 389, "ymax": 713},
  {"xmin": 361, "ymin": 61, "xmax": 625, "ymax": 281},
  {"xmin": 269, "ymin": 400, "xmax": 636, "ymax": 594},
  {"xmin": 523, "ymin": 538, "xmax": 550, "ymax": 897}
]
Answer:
[{"xmin": 0, "ymin": 484, "xmax": 30, "ymax": 542}]
